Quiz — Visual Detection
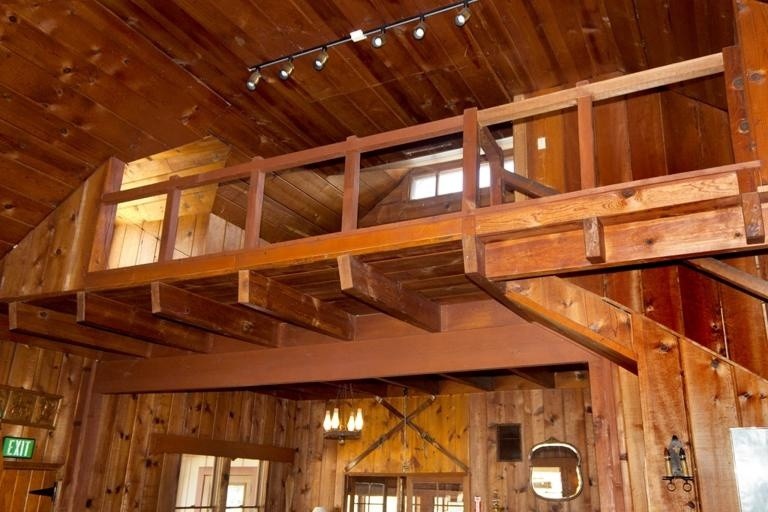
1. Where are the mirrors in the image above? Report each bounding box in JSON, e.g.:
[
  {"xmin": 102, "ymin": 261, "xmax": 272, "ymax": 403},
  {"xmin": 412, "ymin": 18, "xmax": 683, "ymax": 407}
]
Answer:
[{"xmin": 529, "ymin": 436, "xmax": 583, "ymax": 502}]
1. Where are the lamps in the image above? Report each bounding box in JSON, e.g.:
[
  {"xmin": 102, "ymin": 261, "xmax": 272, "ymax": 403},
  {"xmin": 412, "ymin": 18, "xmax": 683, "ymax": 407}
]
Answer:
[
  {"xmin": 323, "ymin": 382, "xmax": 363, "ymax": 439},
  {"xmin": 246, "ymin": 0, "xmax": 478, "ymax": 91},
  {"xmin": 663, "ymin": 435, "xmax": 693, "ymax": 491}
]
